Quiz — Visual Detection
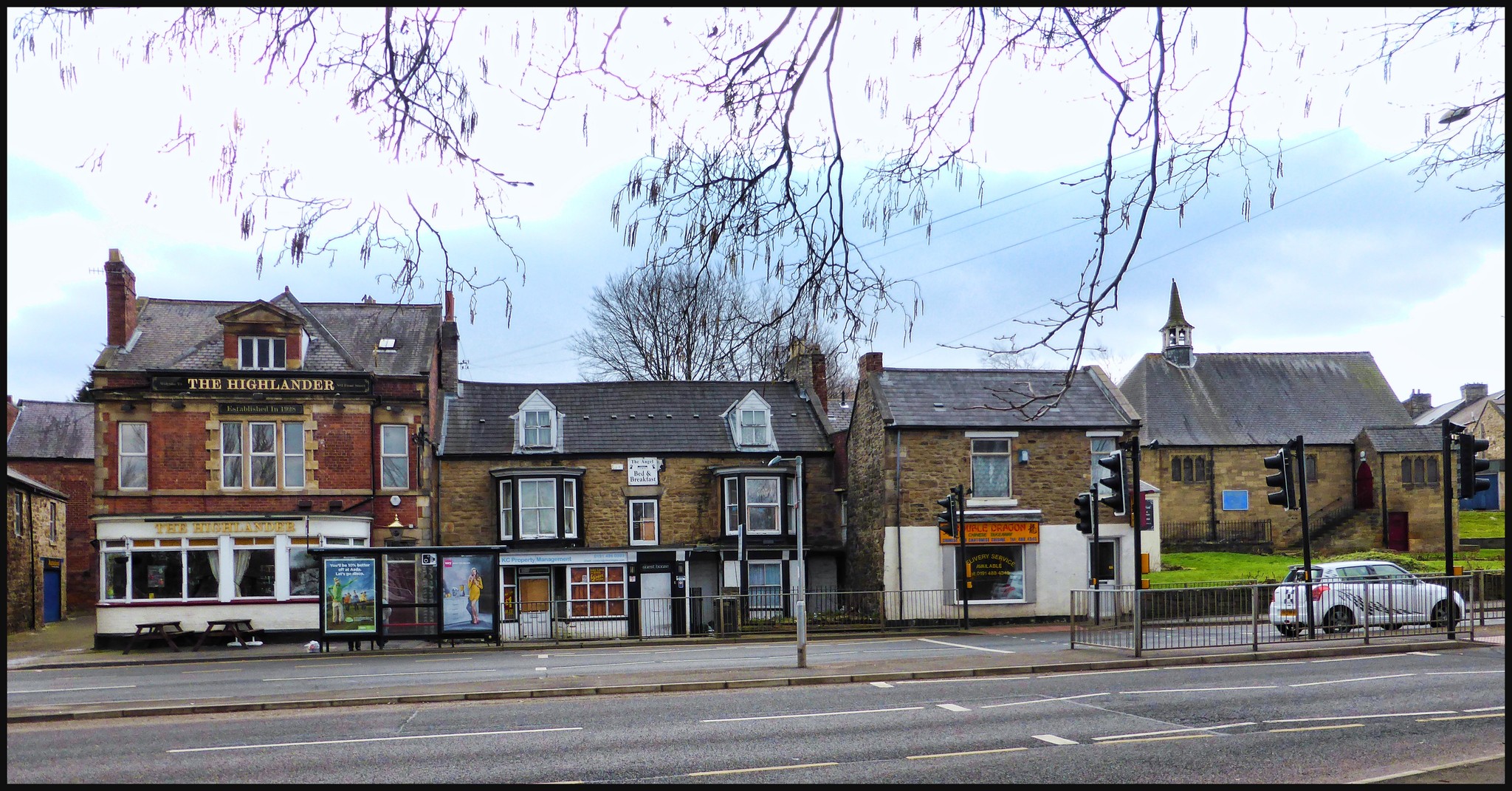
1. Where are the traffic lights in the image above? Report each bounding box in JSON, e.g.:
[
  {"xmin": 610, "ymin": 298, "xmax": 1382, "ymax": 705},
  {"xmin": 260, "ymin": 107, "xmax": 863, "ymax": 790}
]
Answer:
[
  {"xmin": 1264, "ymin": 446, "xmax": 1295, "ymax": 509},
  {"xmin": 937, "ymin": 493, "xmax": 959, "ymax": 537},
  {"xmin": 1097, "ymin": 449, "xmax": 1129, "ymax": 517},
  {"xmin": 1073, "ymin": 493, "xmax": 1094, "ymax": 536},
  {"xmin": 1459, "ymin": 432, "xmax": 1491, "ymax": 498}
]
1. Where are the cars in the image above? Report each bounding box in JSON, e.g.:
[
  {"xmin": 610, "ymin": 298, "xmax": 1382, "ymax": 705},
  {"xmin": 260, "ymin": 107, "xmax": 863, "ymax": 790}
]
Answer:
[{"xmin": 1268, "ymin": 561, "xmax": 1466, "ymax": 636}]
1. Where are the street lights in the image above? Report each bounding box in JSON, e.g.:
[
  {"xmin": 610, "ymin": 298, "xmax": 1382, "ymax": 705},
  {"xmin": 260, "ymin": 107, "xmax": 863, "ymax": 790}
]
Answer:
[{"xmin": 767, "ymin": 454, "xmax": 807, "ymax": 668}]
